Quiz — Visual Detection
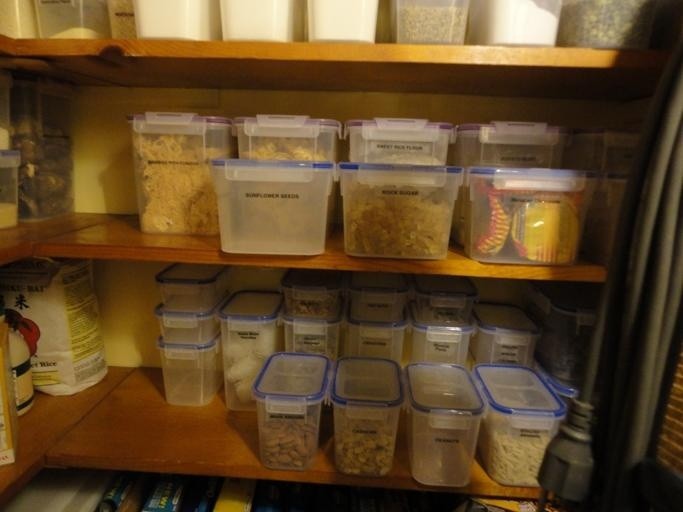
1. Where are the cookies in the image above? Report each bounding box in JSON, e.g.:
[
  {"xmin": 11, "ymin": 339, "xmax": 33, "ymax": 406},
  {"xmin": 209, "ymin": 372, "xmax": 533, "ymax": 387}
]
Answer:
[
  {"xmin": 334, "ymin": 424, "xmax": 393, "ymax": 474},
  {"xmin": 260, "ymin": 418, "xmax": 316, "ymax": 469}
]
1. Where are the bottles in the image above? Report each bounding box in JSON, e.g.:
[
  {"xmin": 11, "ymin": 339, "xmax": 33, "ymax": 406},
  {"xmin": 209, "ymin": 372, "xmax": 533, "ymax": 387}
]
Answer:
[{"xmin": 0, "ymin": 309, "xmax": 35, "ymax": 418}]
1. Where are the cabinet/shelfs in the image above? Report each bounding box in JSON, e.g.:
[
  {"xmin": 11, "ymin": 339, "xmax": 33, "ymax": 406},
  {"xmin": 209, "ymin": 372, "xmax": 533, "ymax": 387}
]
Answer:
[
  {"xmin": 2, "ymin": 25, "xmax": 683, "ymax": 512},
  {"xmin": 0, "ymin": 34, "xmax": 137, "ymax": 512}
]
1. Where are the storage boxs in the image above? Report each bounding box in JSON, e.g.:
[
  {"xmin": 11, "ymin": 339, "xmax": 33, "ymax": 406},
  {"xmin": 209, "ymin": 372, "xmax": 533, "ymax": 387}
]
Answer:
[
  {"xmin": 346, "ymin": 272, "xmax": 409, "ymax": 324},
  {"xmin": 0, "ymin": 461, "xmax": 264, "ymax": 512},
  {"xmin": 471, "ymin": 299, "xmax": 535, "ymax": 368},
  {"xmin": 568, "ymin": 130, "xmax": 646, "ymax": 262},
  {"xmin": 0, "ymin": 66, "xmax": 77, "ymax": 233},
  {"xmin": 215, "ymin": 286, "xmax": 287, "ymax": 414},
  {"xmin": 210, "ymin": 158, "xmax": 337, "ymax": 257},
  {"xmin": 124, "ymin": 111, "xmax": 233, "ymax": 237},
  {"xmin": 410, "ymin": 275, "xmax": 479, "ymax": 327},
  {"xmin": 152, "ymin": 261, "xmax": 232, "ymax": 409},
  {"xmin": 0, "ymin": 0, "xmax": 677, "ymax": 54},
  {"xmin": 474, "ymin": 362, "xmax": 567, "ymax": 488},
  {"xmin": 230, "ymin": 112, "xmax": 343, "ymax": 168},
  {"xmin": 529, "ymin": 279, "xmax": 599, "ymax": 399},
  {"xmin": 336, "ymin": 161, "xmax": 465, "ymax": 261},
  {"xmin": 281, "ymin": 312, "xmax": 343, "ymax": 360},
  {"xmin": 457, "ymin": 163, "xmax": 586, "ymax": 269},
  {"xmin": 401, "ymin": 362, "xmax": 489, "ymax": 487},
  {"xmin": 342, "ymin": 319, "xmax": 410, "ymax": 370},
  {"xmin": 455, "ymin": 119, "xmax": 566, "ymax": 173},
  {"xmin": 343, "ymin": 117, "xmax": 455, "ymax": 167},
  {"xmin": 407, "ymin": 323, "xmax": 480, "ymax": 368},
  {"xmin": 281, "ymin": 269, "xmax": 343, "ymax": 322},
  {"xmin": 248, "ymin": 351, "xmax": 331, "ymax": 473},
  {"xmin": 329, "ymin": 356, "xmax": 406, "ymax": 477}
]
